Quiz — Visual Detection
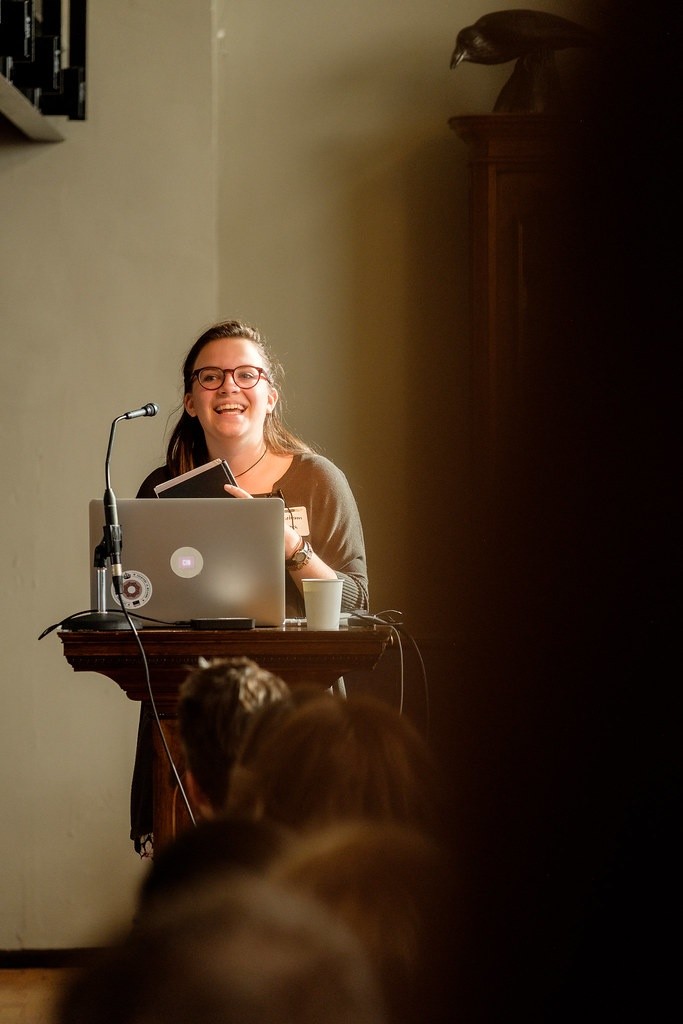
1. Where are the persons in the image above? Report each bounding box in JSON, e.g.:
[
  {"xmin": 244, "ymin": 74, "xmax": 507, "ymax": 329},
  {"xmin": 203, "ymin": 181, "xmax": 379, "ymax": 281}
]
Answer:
[
  {"xmin": 130, "ymin": 320, "xmax": 370, "ymax": 858},
  {"xmin": 55, "ymin": 663, "xmax": 456, "ymax": 1022}
]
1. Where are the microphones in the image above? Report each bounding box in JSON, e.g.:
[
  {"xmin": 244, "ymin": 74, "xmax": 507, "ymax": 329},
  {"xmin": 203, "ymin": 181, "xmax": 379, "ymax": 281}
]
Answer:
[
  {"xmin": 277, "ymin": 489, "xmax": 285, "ymax": 501},
  {"xmin": 103, "ymin": 403, "xmax": 159, "ymax": 594}
]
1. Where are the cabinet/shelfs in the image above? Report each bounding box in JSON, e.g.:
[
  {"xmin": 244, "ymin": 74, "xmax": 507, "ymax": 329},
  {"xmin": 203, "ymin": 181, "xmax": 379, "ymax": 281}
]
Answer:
[{"xmin": 447, "ymin": 110, "xmax": 580, "ymax": 442}]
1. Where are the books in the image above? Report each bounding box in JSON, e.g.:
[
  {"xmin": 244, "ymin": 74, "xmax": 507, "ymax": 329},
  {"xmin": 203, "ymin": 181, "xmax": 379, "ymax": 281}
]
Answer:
[{"xmin": 154, "ymin": 457, "xmax": 238, "ymax": 498}]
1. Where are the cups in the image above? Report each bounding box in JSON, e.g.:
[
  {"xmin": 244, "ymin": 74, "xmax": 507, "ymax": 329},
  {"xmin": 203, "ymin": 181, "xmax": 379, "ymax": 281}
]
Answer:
[{"xmin": 301, "ymin": 578, "xmax": 345, "ymax": 631}]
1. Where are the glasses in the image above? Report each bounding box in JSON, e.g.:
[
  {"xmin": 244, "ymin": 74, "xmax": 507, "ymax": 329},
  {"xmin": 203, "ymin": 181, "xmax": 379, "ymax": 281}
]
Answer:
[{"xmin": 190, "ymin": 365, "xmax": 271, "ymax": 390}]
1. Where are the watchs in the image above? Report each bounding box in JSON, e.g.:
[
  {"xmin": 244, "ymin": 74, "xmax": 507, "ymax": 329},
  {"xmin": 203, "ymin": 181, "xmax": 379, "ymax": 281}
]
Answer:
[{"xmin": 285, "ymin": 541, "xmax": 313, "ymax": 572}]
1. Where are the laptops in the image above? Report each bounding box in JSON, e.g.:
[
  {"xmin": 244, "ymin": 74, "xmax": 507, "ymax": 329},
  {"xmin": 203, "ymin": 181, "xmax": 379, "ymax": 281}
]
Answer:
[{"xmin": 88, "ymin": 498, "xmax": 285, "ymax": 627}]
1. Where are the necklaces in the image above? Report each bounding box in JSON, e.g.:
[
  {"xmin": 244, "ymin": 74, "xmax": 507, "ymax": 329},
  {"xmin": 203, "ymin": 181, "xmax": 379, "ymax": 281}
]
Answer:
[{"xmin": 233, "ymin": 446, "xmax": 268, "ymax": 478}]
